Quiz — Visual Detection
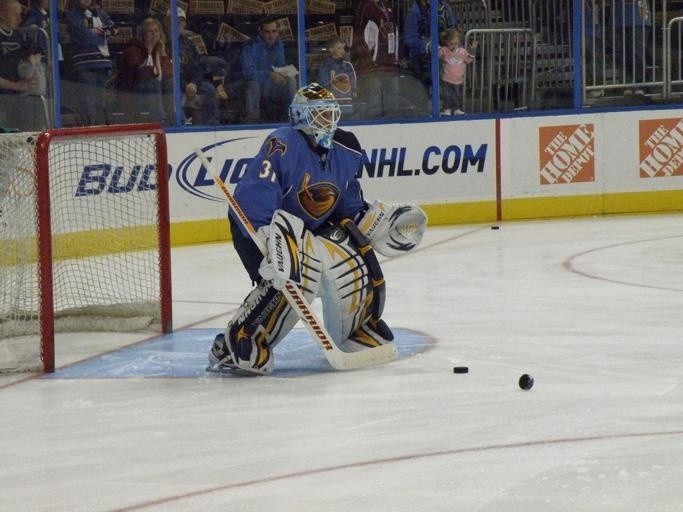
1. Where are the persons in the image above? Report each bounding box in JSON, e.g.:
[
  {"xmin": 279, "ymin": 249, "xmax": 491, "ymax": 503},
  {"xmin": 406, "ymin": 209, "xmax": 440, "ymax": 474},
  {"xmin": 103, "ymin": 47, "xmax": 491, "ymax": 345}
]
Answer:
[
  {"xmin": 203, "ymin": 82, "xmax": 428, "ymax": 377},
  {"xmin": 556, "ymin": 0, "xmax": 653, "ymax": 98},
  {"xmin": 1, "ymin": 1, "xmax": 358, "ymax": 124},
  {"xmin": 356, "ymin": 0, "xmax": 475, "ymax": 116}
]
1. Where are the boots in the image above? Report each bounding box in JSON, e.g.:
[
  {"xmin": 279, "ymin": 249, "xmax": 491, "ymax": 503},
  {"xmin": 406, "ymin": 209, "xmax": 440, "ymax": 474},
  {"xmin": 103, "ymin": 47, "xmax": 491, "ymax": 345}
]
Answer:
[{"xmin": 208, "ymin": 334, "xmax": 235, "ymax": 368}]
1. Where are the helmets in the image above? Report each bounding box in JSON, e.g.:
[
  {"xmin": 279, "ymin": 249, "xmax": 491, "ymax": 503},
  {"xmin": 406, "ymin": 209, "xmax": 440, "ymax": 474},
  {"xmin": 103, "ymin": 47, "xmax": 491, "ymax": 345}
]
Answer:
[{"xmin": 288, "ymin": 82, "xmax": 341, "ymax": 130}]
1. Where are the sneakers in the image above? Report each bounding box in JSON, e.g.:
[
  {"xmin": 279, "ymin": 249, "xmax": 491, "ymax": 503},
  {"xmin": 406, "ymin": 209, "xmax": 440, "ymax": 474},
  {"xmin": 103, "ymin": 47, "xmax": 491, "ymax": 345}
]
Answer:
[{"xmin": 440, "ymin": 109, "xmax": 465, "ymax": 116}]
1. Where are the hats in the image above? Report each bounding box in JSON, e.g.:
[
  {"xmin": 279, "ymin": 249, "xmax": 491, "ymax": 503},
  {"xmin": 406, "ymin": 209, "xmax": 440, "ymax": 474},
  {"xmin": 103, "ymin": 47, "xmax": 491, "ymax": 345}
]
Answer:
[{"xmin": 166, "ymin": 7, "xmax": 186, "ymax": 20}]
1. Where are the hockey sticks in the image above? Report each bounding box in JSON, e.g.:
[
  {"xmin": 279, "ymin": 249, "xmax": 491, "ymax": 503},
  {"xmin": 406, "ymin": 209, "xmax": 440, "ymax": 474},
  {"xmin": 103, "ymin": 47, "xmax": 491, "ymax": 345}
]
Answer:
[{"xmin": 193, "ymin": 145, "xmax": 399, "ymax": 371}]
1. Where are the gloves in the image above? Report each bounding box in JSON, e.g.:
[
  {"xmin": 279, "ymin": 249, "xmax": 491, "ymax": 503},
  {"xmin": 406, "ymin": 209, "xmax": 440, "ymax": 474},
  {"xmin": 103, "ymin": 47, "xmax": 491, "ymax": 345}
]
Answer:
[
  {"xmin": 258, "ymin": 210, "xmax": 304, "ymax": 290},
  {"xmin": 352, "ymin": 202, "xmax": 428, "ymax": 258}
]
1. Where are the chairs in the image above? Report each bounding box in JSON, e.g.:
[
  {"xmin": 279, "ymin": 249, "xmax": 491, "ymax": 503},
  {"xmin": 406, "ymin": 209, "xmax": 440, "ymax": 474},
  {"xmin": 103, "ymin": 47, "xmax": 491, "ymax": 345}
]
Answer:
[{"xmin": 109, "ymin": 13, "xmax": 340, "ymax": 83}]
